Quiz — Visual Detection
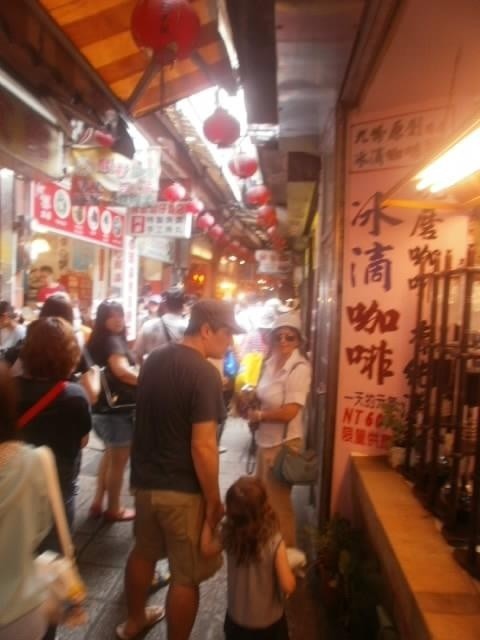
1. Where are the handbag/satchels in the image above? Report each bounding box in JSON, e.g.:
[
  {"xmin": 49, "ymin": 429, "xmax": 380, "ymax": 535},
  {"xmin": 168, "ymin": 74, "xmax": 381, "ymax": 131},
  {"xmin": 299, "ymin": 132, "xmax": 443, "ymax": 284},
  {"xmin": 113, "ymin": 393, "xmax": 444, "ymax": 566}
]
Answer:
[
  {"xmin": 234, "ymin": 352, "xmax": 262, "ymax": 390},
  {"xmin": 224, "ymin": 351, "xmax": 238, "ymax": 376},
  {"xmin": 34, "ymin": 550, "xmax": 89, "ymax": 626},
  {"xmin": 271, "ymin": 444, "xmax": 319, "ymax": 484},
  {"xmin": 91, "ymin": 365, "xmax": 139, "ymax": 413}
]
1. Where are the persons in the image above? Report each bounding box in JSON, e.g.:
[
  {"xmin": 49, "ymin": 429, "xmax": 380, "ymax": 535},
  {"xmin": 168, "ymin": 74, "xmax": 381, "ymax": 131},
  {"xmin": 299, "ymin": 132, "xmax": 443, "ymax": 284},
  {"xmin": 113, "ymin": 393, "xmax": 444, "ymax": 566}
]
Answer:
[{"xmin": 1, "ymin": 265, "xmax": 314, "ymax": 639}]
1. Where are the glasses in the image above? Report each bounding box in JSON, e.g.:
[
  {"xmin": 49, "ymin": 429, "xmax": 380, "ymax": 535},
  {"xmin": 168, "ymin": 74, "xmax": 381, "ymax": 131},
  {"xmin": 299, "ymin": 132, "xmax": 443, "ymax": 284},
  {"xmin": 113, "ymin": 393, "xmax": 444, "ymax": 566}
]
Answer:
[
  {"xmin": 271, "ymin": 334, "xmax": 297, "ymax": 342},
  {"xmin": 104, "ymin": 298, "xmax": 121, "ymax": 305}
]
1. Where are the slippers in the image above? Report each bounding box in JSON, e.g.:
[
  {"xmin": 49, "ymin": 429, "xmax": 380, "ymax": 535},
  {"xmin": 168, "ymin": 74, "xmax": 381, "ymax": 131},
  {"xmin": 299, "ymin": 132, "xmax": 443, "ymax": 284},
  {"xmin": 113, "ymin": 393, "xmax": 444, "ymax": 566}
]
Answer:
[
  {"xmin": 149, "ymin": 559, "xmax": 170, "ymax": 591},
  {"xmin": 116, "ymin": 604, "xmax": 165, "ymax": 638}
]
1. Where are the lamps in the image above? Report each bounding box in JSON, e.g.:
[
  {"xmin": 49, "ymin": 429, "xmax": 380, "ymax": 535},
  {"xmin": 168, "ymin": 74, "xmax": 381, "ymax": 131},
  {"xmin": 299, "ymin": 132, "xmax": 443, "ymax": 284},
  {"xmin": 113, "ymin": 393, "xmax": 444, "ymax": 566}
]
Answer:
[{"xmin": 410, "ymin": 115, "xmax": 480, "ymax": 194}]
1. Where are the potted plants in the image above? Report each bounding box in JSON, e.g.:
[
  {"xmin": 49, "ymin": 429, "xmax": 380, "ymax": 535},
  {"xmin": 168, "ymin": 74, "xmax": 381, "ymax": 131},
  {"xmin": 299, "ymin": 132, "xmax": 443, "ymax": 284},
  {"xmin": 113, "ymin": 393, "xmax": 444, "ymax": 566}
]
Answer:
[{"xmin": 384, "ymin": 399, "xmax": 416, "ymax": 469}]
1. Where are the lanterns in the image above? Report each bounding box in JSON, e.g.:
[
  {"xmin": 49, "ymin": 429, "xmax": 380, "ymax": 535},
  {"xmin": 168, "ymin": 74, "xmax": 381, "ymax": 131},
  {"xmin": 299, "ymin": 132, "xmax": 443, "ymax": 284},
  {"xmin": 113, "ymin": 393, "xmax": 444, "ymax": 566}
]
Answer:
[
  {"xmin": 207, "ymin": 223, "xmax": 224, "ymax": 240},
  {"xmin": 202, "ymin": 107, "xmax": 242, "ymax": 149},
  {"xmin": 185, "ymin": 198, "xmax": 205, "ymax": 215},
  {"xmin": 129, "ymin": 0, "xmax": 203, "ymax": 70},
  {"xmin": 163, "ymin": 182, "xmax": 188, "ymax": 204},
  {"xmin": 95, "ymin": 128, "xmax": 119, "ymax": 148},
  {"xmin": 211, "ymin": 206, "xmax": 295, "ymax": 273},
  {"xmin": 240, "ymin": 183, "xmax": 271, "ymax": 209},
  {"xmin": 226, "ymin": 150, "xmax": 260, "ymax": 197},
  {"xmin": 193, "ymin": 212, "xmax": 214, "ymax": 231}
]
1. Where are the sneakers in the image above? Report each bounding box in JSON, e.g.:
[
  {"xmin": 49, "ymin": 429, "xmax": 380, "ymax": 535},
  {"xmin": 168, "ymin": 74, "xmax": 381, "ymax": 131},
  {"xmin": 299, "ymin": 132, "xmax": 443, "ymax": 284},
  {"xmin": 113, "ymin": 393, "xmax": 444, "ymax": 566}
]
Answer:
[
  {"xmin": 89, "ymin": 503, "xmax": 102, "ymax": 517},
  {"xmin": 105, "ymin": 506, "xmax": 135, "ymax": 521}
]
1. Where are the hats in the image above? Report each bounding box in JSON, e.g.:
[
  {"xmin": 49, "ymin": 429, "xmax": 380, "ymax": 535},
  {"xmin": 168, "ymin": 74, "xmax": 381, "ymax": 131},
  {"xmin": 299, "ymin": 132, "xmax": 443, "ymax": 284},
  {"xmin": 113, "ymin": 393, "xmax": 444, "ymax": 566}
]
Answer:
[
  {"xmin": 266, "ymin": 299, "xmax": 288, "ymax": 312},
  {"xmin": 256, "ymin": 308, "xmax": 277, "ymax": 328},
  {"xmin": 265, "ymin": 314, "xmax": 307, "ymax": 346},
  {"xmin": 192, "ymin": 301, "xmax": 247, "ymax": 334},
  {"xmin": 144, "ymin": 295, "xmax": 166, "ymax": 308},
  {"xmin": 162, "ymin": 287, "xmax": 182, "ymax": 299}
]
